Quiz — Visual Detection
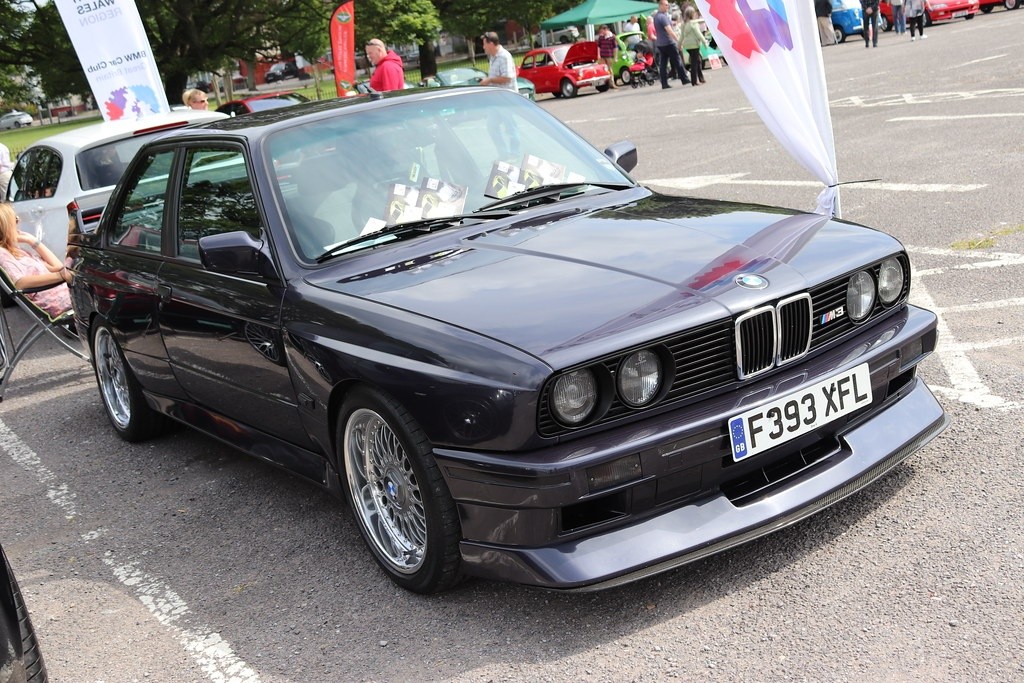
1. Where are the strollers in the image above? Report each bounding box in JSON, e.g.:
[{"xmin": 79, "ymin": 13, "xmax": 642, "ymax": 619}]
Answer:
[{"xmin": 628, "ymin": 40, "xmax": 662, "ymax": 88}]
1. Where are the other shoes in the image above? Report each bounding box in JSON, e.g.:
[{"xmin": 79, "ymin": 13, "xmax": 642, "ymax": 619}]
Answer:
[
  {"xmin": 911, "ymin": 37, "xmax": 915, "ymax": 41},
  {"xmin": 921, "ymin": 35, "xmax": 928, "ymax": 39},
  {"xmin": 896, "ymin": 32, "xmax": 907, "ymax": 35},
  {"xmin": 610, "ymin": 84, "xmax": 617, "ymax": 89}
]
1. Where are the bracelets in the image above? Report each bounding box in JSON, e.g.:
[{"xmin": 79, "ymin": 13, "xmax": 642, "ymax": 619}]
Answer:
[{"xmin": 32, "ymin": 239, "xmax": 42, "ymax": 250}]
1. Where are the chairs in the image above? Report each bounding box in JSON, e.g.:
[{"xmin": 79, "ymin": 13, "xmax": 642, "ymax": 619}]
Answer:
[{"xmin": 0, "ymin": 264, "xmax": 91, "ymax": 403}]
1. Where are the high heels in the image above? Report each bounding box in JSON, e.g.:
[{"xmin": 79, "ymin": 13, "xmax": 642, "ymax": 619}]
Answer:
[
  {"xmin": 700, "ymin": 80, "xmax": 706, "ymax": 83},
  {"xmin": 693, "ymin": 83, "xmax": 699, "ymax": 86}
]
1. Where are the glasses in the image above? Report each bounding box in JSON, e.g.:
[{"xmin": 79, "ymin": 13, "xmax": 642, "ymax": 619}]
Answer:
[
  {"xmin": 365, "ymin": 40, "xmax": 381, "ymax": 46},
  {"xmin": 664, "ymin": 4, "xmax": 669, "ymax": 6},
  {"xmin": 482, "ymin": 32, "xmax": 494, "ymax": 43},
  {"xmin": 16, "ymin": 216, "xmax": 19, "ymax": 224},
  {"xmin": 192, "ymin": 99, "xmax": 209, "ymax": 104}
]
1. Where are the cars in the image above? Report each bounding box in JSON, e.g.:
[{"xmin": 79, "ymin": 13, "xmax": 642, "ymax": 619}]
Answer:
[
  {"xmin": 515, "ymin": 41, "xmax": 612, "ymax": 97},
  {"xmin": 878, "ymin": 0, "xmax": 980, "ymax": 32},
  {"xmin": 0, "ymin": 112, "xmax": 34, "ymax": 129},
  {"xmin": 0, "ymin": 108, "xmax": 298, "ymax": 307},
  {"xmin": 353, "ymin": 79, "xmax": 455, "ymax": 126},
  {"xmin": 65, "ymin": 85, "xmax": 952, "ymax": 600},
  {"xmin": 678, "ymin": 20, "xmax": 729, "ymax": 71},
  {"xmin": 213, "ymin": 89, "xmax": 342, "ymax": 183},
  {"xmin": 264, "ymin": 62, "xmax": 298, "ymax": 85},
  {"xmin": 216, "ymin": 75, "xmax": 248, "ymax": 92},
  {"xmin": 827, "ymin": 0, "xmax": 883, "ymax": 43},
  {"xmin": 436, "ymin": 67, "xmax": 536, "ymax": 103},
  {"xmin": 977, "ymin": 0, "xmax": 1024, "ymax": 14},
  {"xmin": 595, "ymin": 31, "xmax": 653, "ymax": 86}
]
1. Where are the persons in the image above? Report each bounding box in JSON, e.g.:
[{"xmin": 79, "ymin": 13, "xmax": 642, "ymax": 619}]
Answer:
[
  {"xmin": 815, "ymin": 0, "xmax": 836, "ymax": 46},
  {"xmin": 0, "ymin": 144, "xmax": 13, "ymax": 201},
  {"xmin": 366, "ymin": 39, "xmax": 405, "ymax": 94},
  {"xmin": 906, "ymin": 1, "xmax": 933, "ymax": 42},
  {"xmin": 0, "ymin": 202, "xmax": 73, "ymax": 319},
  {"xmin": 597, "ymin": 0, "xmax": 710, "ymax": 90},
  {"xmin": 886, "ymin": 0, "xmax": 906, "ymax": 36},
  {"xmin": 183, "ymin": 89, "xmax": 209, "ymax": 111},
  {"xmin": 480, "ymin": 31, "xmax": 519, "ymax": 93},
  {"xmin": 560, "ymin": 35, "xmax": 571, "ymax": 44},
  {"xmin": 859, "ymin": 0, "xmax": 880, "ymax": 47}
]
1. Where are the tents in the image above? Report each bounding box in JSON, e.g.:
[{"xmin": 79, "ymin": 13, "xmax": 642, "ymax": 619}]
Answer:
[{"xmin": 540, "ymin": 0, "xmax": 660, "ymax": 48}]
